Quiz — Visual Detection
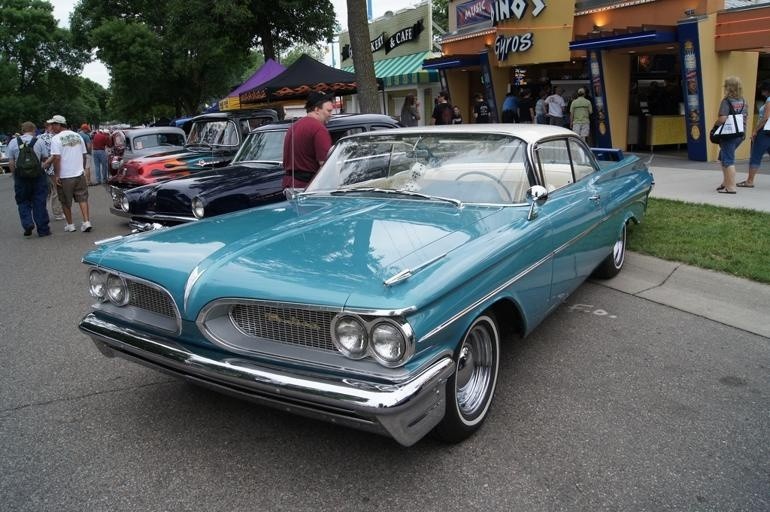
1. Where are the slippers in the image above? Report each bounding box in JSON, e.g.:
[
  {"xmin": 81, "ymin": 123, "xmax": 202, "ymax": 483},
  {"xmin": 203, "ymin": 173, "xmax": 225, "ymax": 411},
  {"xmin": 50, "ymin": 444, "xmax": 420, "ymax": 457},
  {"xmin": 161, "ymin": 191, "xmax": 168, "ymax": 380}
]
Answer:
[{"xmin": 717, "ymin": 181, "xmax": 753, "ymax": 193}]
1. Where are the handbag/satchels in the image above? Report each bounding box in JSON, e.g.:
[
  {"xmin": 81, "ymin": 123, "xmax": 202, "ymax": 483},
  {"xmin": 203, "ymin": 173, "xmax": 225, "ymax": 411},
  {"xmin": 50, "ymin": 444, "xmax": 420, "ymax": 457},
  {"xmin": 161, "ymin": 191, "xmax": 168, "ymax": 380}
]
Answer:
[{"xmin": 710, "ymin": 113, "xmax": 746, "ymax": 143}]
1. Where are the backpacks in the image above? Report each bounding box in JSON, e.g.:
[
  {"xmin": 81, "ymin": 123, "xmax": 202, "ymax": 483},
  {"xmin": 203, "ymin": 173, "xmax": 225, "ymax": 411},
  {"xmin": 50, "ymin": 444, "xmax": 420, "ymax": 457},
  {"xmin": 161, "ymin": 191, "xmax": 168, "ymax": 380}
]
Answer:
[{"xmin": 17, "ymin": 136, "xmax": 42, "ymax": 178}]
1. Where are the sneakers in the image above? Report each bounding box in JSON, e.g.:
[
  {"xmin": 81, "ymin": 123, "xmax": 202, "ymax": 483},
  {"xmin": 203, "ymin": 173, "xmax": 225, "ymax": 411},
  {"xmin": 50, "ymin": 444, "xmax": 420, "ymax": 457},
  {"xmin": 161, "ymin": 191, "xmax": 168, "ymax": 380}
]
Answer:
[
  {"xmin": 24, "ymin": 225, "xmax": 34, "ymax": 235},
  {"xmin": 64, "ymin": 224, "xmax": 76, "ymax": 231},
  {"xmin": 81, "ymin": 221, "xmax": 92, "ymax": 232}
]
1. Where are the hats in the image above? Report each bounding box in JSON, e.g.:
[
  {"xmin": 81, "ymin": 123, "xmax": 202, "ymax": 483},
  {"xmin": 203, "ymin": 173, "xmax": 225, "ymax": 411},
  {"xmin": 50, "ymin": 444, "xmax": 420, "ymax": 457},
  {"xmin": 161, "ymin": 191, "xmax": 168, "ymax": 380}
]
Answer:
[
  {"xmin": 47, "ymin": 115, "xmax": 66, "ymax": 124},
  {"xmin": 81, "ymin": 124, "xmax": 90, "ymax": 130},
  {"xmin": 307, "ymin": 91, "xmax": 333, "ymax": 106}
]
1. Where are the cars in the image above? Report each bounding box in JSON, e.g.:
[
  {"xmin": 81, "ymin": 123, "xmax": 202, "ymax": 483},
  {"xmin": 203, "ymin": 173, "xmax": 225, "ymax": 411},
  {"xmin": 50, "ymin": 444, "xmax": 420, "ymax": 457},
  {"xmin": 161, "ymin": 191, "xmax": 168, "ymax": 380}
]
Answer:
[
  {"xmin": 111, "ymin": 125, "xmax": 188, "ymax": 180},
  {"xmin": 77, "ymin": 123, "xmax": 655, "ymax": 451},
  {"xmin": 121, "ymin": 112, "xmax": 399, "ymax": 236},
  {"xmin": 111, "ymin": 107, "xmax": 282, "ymax": 219}
]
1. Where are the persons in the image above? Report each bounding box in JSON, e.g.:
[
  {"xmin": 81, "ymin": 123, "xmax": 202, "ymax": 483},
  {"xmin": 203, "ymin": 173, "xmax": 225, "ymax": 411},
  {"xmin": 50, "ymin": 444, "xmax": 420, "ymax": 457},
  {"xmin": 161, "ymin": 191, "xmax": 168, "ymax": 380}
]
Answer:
[
  {"xmin": 716, "ymin": 76, "xmax": 749, "ymax": 194},
  {"xmin": 400, "ymin": 94, "xmax": 420, "ymax": 127},
  {"xmin": 501, "ymin": 88, "xmax": 593, "ymax": 143},
  {"xmin": 736, "ymin": 79, "xmax": 770, "ymax": 187},
  {"xmin": 432, "ymin": 92, "xmax": 453, "ymax": 127},
  {"xmin": 472, "ymin": 92, "xmax": 491, "ymax": 123},
  {"xmin": 5, "ymin": 121, "xmax": 51, "ymax": 237},
  {"xmin": 454, "ymin": 106, "xmax": 464, "ymax": 124},
  {"xmin": 282, "ymin": 94, "xmax": 333, "ymax": 191},
  {"xmin": 47, "ymin": 114, "xmax": 91, "ymax": 232},
  {"xmin": 37, "ymin": 120, "xmax": 66, "ymax": 220},
  {"xmin": 12, "ymin": 124, "xmax": 113, "ymax": 186}
]
